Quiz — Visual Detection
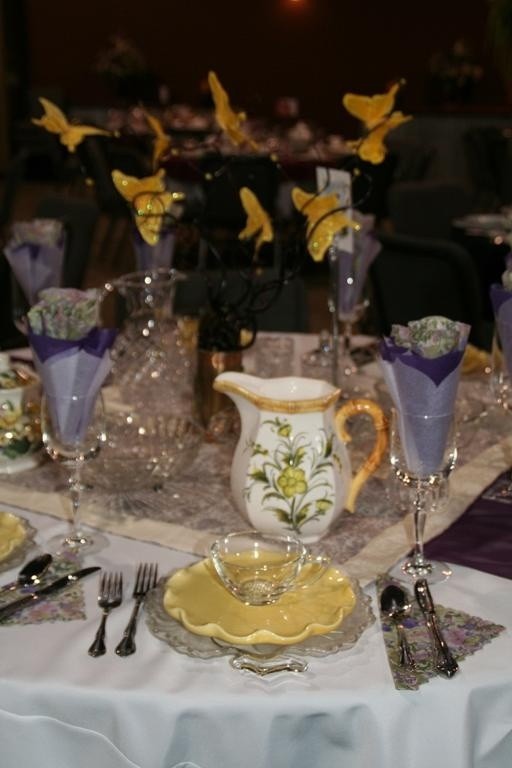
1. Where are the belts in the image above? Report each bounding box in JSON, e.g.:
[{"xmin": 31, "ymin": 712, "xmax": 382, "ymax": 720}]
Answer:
[
  {"xmin": 488, "ymin": 319, "xmax": 512, "ymax": 501},
  {"xmin": 325, "ymin": 264, "xmax": 377, "ymax": 375},
  {"xmin": 41, "ymin": 391, "xmax": 110, "ymax": 559},
  {"xmin": 389, "ymin": 403, "xmax": 459, "ymax": 591}
]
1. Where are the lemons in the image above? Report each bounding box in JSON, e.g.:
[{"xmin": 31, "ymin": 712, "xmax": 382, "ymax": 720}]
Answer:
[{"xmin": 211, "ymin": 369, "xmax": 387, "ymax": 545}]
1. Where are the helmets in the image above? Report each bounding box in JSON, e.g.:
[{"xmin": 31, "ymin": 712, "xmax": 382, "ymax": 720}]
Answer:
[{"xmin": 163, "ymin": 553, "xmax": 363, "ymax": 648}]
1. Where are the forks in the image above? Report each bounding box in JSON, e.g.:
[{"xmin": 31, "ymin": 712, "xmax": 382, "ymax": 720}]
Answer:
[{"xmin": 87, "ymin": 563, "xmax": 158, "ymax": 658}]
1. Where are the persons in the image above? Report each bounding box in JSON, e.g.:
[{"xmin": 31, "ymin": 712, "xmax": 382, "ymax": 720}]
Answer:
[{"xmin": 2, "ymin": 33, "xmax": 511, "ymax": 159}]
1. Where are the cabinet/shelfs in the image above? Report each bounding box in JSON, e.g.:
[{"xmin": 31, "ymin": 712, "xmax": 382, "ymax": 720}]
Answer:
[{"xmin": 88, "ymin": 270, "xmax": 205, "ymax": 493}]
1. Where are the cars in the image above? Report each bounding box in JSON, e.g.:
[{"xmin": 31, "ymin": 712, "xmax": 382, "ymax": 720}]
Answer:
[{"xmin": 211, "ymin": 528, "xmax": 306, "ymax": 609}]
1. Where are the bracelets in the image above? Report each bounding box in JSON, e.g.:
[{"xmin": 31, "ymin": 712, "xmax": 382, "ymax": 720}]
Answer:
[{"xmin": 0, "ymin": 363, "xmax": 40, "ymax": 413}]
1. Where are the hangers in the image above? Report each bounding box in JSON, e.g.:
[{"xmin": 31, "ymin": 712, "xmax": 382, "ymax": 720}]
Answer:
[
  {"xmin": 379, "ymin": 581, "xmax": 414, "ymax": 675},
  {"xmin": 0, "ymin": 551, "xmax": 53, "ymax": 597}
]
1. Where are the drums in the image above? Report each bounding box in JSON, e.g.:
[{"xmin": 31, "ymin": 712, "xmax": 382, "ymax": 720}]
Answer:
[
  {"xmin": 2, "ymin": 560, "xmax": 100, "ymax": 629},
  {"xmin": 405, "ymin": 580, "xmax": 463, "ymax": 676}
]
